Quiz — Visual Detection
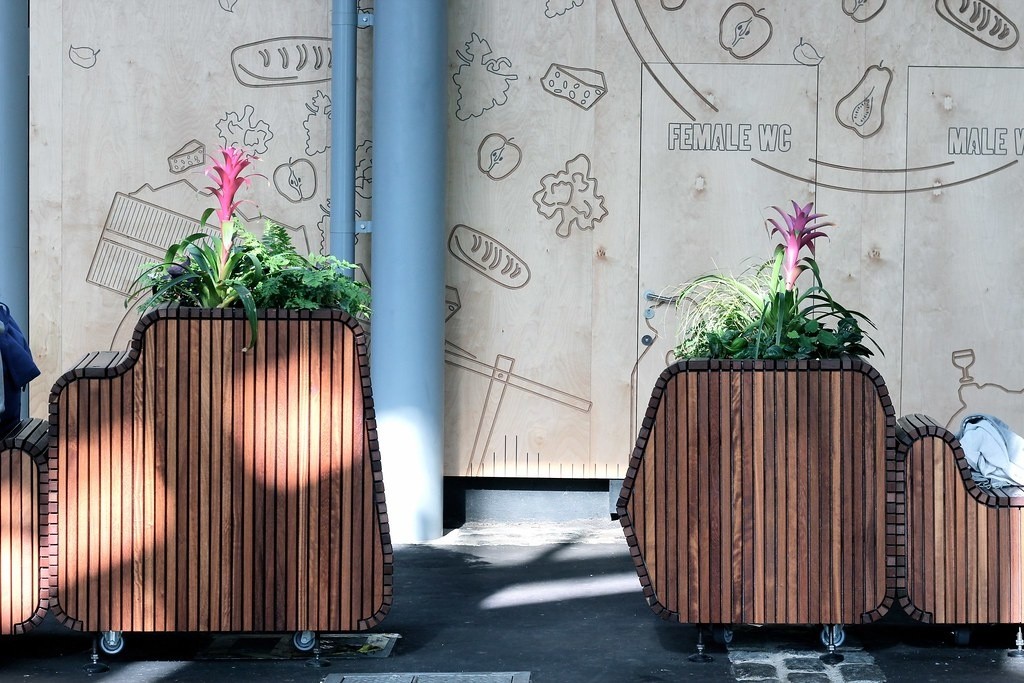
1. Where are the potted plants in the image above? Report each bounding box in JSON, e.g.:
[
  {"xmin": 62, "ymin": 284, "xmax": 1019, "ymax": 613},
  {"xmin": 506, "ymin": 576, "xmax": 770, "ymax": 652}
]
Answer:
[
  {"xmin": 47, "ymin": 142, "xmax": 392, "ymax": 652},
  {"xmin": 614, "ymin": 213, "xmax": 897, "ymax": 647}
]
